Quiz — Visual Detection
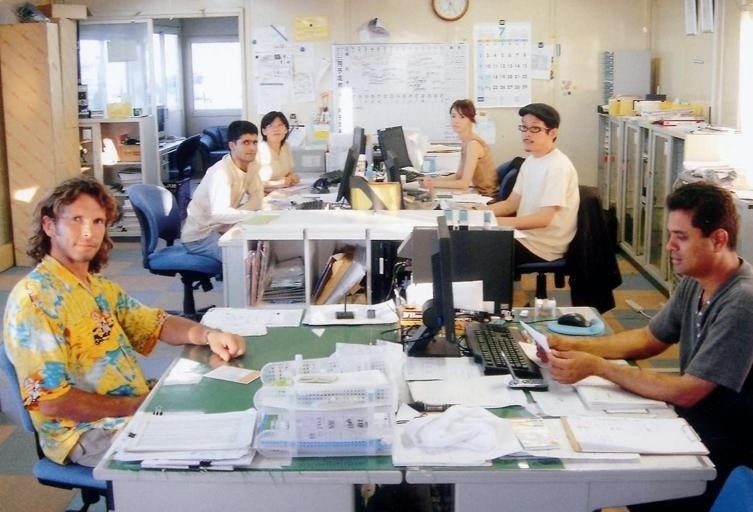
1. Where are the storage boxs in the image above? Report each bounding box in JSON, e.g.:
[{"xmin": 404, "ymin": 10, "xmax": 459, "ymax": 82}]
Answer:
[{"xmin": 36, "ymin": 2, "xmax": 93, "ymax": 20}]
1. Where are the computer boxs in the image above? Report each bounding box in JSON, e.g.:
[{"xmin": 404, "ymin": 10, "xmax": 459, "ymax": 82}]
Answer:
[
  {"xmin": 410, "ymin": 226, "xmax": 514, "ymax": 314},
  {"xmin": 371, "ymin": 240, "xmax": 394, "ymax": 303}
]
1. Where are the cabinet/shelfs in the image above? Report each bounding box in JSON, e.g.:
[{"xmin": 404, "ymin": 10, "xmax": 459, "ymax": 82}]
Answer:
[
  {"xmin": 597, "ymin": 112, "xmax": 752, "ymax": 297},
  {"xmin": 76, "ymin": 115, "xmax": 163, "ymax": 240}
]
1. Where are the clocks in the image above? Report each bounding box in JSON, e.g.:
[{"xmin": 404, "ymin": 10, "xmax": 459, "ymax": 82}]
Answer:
[{"xmin": 432, "ymin": 0, "xmax": 468, "ymax": 21}]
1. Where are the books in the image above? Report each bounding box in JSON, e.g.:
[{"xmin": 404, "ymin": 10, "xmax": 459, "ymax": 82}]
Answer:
[
  {"xmin": 605, "ymin": 90, "xmax": 712, "ymax": 132},
  {"xmin": 106, "ymin": 170, "xmax": 146, "ymax": 233},
  {"xmin": 400, "ymin": 352, "xmax": 712, "ymax": 466},
  {"xmin": 676, "ymin": 161, "xmax": 751, "ymax": 193},
  {"xmin": 244, "ymin": 240, "xmax": 365, "ymax": 306},
  {"xmin": 113, "ymin": 403, "xmax": 255, "ymax": 467}
]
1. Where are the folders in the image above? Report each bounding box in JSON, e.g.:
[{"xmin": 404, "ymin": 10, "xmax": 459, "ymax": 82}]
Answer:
[{"xmin": 651, "ymin": 120, "xmax": 705, "ymax": 126}]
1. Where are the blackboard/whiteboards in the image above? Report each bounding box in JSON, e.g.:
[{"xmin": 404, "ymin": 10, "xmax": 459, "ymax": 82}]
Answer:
[{"xmin": 331, "ymin": 43, "xmax": 468, "ymax": 145}]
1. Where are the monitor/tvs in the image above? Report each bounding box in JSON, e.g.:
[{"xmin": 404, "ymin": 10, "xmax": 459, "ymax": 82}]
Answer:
[
  {"xmin": 385, "ymin": 150, "xmax": 406, "ymax": 209},
  {"xmin": 352, "ymin": 125, "xmax": 367, "ymax": 154},
  {"xmin": 406, "ymin": 216, "xmax": 461, "ymax": 359},
  {"xmin": 378, "ymin": 125, "xmax": 413, "ymax": 174},
  {"xmin": 335, "ymin": 144, "xmax": 360, "ymax": 210}
]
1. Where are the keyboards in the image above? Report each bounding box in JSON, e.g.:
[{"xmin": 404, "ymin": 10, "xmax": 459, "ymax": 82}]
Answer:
[
  {"xmin": 321, "ymin": 170, "xmax": 345, "ymax": 183},
  {"xmin": 399, "ymin": 169, "xmax": 423, "ymax": 183},
  {"xmin": 402, "ymin": 170, "xmax": 417, "ymax": 182},
  {"xmin": 296, "ymin": 200, "xmax": 322, "ymax": 209},
  {"xmin": 464, "ymin": 323, "xmax": 543, "ymax": 379}
]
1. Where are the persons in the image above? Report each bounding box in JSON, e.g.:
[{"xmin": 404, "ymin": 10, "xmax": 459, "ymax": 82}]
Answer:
[
  {"xmin": 2, "ymin": 178, "xmax": 245, "ymax": 469},
  {"xmin": 256, "ymin": 112, "xmax": 301, "ymax": 194},
  {"xmin": 421, "ymin": 99, "xmax": 499, "ymax": 200},
  {"xmin": 179, "ymin": 121, "xmax": 264, "ymax": 264},
  {"xmin": 536, "ymin": 183, "xmax": 753, "ymax": 511},
  {"xmin": 470, "ymin": 105, "xmax": 580, "ymax": 265}
]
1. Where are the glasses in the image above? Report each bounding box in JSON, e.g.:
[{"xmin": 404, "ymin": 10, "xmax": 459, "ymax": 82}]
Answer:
[{"xmin": 519, "ymin": 125, "xmax": 548, "ymax": 133}]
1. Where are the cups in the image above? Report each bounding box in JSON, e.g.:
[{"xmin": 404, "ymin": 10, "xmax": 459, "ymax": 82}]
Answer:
[{"xmin": 534, "ymin": 299, "xmax": 556, "ymax": 327}]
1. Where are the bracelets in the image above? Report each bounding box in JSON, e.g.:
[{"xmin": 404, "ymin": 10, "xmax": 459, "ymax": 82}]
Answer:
[{"xmin": 206, "ymin": 327, "xmax": 219, "ymax": 343}]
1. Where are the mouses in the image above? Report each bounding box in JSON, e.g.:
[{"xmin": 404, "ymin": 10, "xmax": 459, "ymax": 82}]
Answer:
[
  {"xmin": 558, "ymin": 313, "xmax": 591, "ymax": 327},
  {"xmin": 315, "ymin": 184, "xmax": 328, "ymax": 190}
]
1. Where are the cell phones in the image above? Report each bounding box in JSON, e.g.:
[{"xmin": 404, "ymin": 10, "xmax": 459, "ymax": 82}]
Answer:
[{"xmin": 500, "ymin": 351, "xmax": 547, "ymax": 389}]
